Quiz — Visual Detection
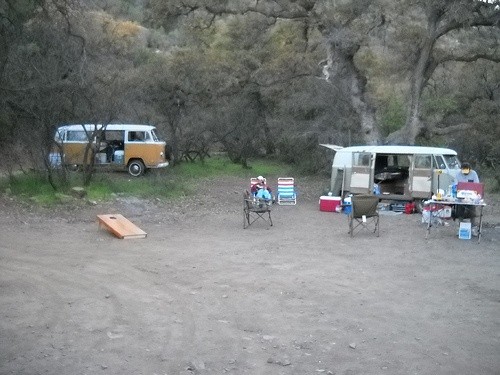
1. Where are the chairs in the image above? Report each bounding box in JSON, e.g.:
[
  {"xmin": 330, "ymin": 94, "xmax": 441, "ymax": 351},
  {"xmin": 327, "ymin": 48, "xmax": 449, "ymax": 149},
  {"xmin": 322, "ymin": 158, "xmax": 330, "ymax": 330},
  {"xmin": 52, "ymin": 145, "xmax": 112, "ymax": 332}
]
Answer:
[
  {"xmin": 348, "ymin": 194, "xmax": 379, "ymax": 239},
  {"xmin": 250, "ymin": 177, "xmax": 267, "ymax": 206},
  {"xmin": 242, "ymin": 189, "xmax": 273, "ymax": 230},
  {"xmin": 277, "ymin": 176, "xmax": 296, "ymax": 206}
]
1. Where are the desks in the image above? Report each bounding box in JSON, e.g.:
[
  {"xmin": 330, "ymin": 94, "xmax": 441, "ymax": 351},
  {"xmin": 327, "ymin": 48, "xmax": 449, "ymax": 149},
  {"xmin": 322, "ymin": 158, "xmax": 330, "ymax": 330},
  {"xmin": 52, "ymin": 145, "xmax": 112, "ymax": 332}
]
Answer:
[{"xmin": 425, "ymin": 199, "xmax": 486, "ymax": 243}]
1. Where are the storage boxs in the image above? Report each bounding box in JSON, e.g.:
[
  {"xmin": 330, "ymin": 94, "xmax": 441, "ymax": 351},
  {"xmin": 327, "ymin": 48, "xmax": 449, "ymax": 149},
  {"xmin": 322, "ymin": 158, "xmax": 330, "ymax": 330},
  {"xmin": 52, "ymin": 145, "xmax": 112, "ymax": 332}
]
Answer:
[
  {"xmin": 113, "ymin": 149, "xmax": 124, "ymax": 163},
  {"xmin": 452, "ymin": 185, "xmax": 458, "ymax": 198},
  {"xmin": 457, "ymin": 182, "xmax": 485, "ymax": 200},
  {"xmin": 95, "ymin": 152, "xmax": 108, "ymax": 163},
  {"xmin": 459, "ymin": 221, "xmax": 472, "ymax": 240},
  {"xmin": 421, "ymin": 207, "xmax": 439, "ymax": 225},
  {"xmin": 343, "ymin": 196, "xmax": 353, "ymax": 214},
  {"xmin": 438, "ymin": 204, "xmax": 451, "ymax": 219},
  {"xmin": 318, "ymin": 195, "xmax": 342, "ymax": 212}
]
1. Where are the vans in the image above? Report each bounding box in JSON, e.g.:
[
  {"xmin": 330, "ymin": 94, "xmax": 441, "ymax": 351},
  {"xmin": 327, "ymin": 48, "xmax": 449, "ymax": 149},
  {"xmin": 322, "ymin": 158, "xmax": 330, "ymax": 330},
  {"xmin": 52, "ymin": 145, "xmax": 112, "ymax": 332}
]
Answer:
[
  {"xmin": 49, "ymin": 125, "xmax": 169, "ymax": 179},
  {"xmin": 319, "ymin": 144, "xmax": 462, "ymax": 214}
]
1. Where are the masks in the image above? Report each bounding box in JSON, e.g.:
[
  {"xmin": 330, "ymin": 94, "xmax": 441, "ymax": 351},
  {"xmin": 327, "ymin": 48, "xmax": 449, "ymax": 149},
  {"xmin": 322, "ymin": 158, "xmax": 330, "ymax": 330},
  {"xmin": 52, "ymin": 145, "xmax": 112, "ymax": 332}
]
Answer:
[{"xmin": 462, "ymin": 169, "xmax": 469, "ymax": 174}]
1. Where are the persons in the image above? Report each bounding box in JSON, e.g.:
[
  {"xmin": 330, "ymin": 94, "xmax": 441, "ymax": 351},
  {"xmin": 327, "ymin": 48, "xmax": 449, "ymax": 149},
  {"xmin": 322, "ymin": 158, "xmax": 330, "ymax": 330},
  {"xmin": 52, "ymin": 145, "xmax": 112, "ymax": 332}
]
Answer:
[
  {"xmin": 251, "ymin": 175, "xmax": 271, "ymax": 208},
  {"xmin": 454, "ymin": 162, "xmax": 479, "ymax": 236}
]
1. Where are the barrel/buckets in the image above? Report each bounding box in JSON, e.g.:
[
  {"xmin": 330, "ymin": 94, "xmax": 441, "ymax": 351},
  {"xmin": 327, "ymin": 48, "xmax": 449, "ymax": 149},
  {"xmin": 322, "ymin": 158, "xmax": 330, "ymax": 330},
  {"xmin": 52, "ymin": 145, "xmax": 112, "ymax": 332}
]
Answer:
[{"xmin": 318, "ymin": 195, "xmax": 351, "ymax": 213}]
